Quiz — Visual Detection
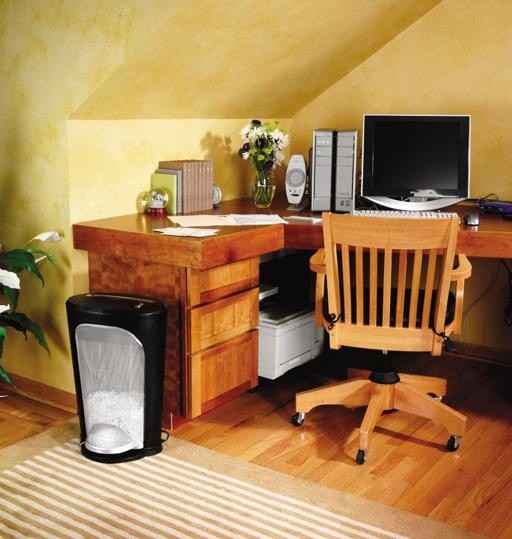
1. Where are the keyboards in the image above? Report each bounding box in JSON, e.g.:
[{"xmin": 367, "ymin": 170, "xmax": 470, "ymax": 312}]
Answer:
[{"xmin": 351, "ymin": 209, "xmax": 460, "ymax": 225}]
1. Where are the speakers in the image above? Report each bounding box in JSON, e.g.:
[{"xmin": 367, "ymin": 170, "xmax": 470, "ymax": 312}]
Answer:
[{"xmin": 285, "ymin": 154, "xmax": 308, "ymax": 212}]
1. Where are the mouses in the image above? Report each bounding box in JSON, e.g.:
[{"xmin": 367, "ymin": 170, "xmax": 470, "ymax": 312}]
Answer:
[{"xmin": 465, "ymin": 213, "xmax": 479, "ymax": 226}]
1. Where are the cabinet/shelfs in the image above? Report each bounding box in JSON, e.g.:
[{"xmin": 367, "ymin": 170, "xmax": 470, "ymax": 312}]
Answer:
[{"xmin": 87, "ymin": 252, "xmax": 259, "ymax": 420}]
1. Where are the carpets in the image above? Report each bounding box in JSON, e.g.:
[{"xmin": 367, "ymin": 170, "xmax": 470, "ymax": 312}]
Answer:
[{"xmin": 0, "ymin": 416, "xmax": 493, "ymax": 539}]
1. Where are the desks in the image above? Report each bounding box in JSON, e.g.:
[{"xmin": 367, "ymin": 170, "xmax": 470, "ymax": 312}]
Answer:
[{"xmin": 72, "ymin": 198, "xmax": 511, "ymax": 271}]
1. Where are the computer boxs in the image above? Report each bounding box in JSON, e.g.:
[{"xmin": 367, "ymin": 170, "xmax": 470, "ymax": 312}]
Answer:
[{"xmin": 311, "ymin": 128, "xmax": 357, "ymax": 212}]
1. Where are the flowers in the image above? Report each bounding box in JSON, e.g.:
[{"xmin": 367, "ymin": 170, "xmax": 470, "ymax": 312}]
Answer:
[{"xmin": 238, "ymin": 119, "xmax": 290, "ymax": 208}]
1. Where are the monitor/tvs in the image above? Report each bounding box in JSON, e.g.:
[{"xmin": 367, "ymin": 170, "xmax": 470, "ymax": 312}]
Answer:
[{"xmin": 361, "ymin": 114, "xmax": 472, "ymax": 211}]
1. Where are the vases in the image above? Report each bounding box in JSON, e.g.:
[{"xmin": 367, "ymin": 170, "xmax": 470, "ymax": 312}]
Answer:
[{"xmin": 252, "ymin": 170, "xmax": 276, "ymax": 209}]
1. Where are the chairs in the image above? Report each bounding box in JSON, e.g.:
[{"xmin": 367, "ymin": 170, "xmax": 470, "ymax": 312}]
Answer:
[{"xmin": 290, "ymin": 211, "xmax": 473, "ymax": 465}]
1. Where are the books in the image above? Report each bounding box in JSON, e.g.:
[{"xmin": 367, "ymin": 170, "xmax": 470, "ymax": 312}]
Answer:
[{"xmin": 150, "ymin": 159, "xmax": 214, "ymax": 217}]
